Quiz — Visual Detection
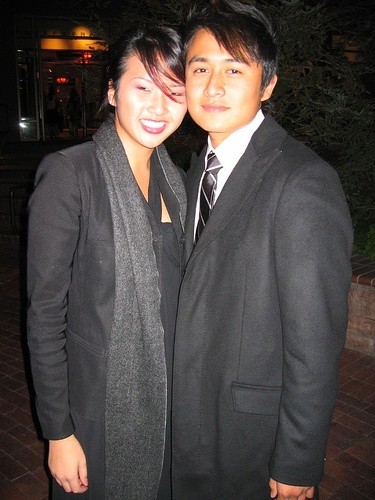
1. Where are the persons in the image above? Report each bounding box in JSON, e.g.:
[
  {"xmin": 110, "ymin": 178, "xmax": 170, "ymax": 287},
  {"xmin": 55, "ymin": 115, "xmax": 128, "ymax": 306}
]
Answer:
[
  {"xmin": 25, "ymin": 22, "xmax": 188, "ymax": 500},
  {"xmin": 47, "ymin": 85, "xmax": 80, "ymax": 135},
  {"xmin": 171, "ymin": 0, "xmax": 353, "ymax": 500}
]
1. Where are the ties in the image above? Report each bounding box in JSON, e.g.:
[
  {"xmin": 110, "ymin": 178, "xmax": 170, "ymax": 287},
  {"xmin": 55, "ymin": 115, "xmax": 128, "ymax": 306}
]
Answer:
[{"xmin": 195, "ymin": 151, "xmax": 223, "ymax": 244}]
